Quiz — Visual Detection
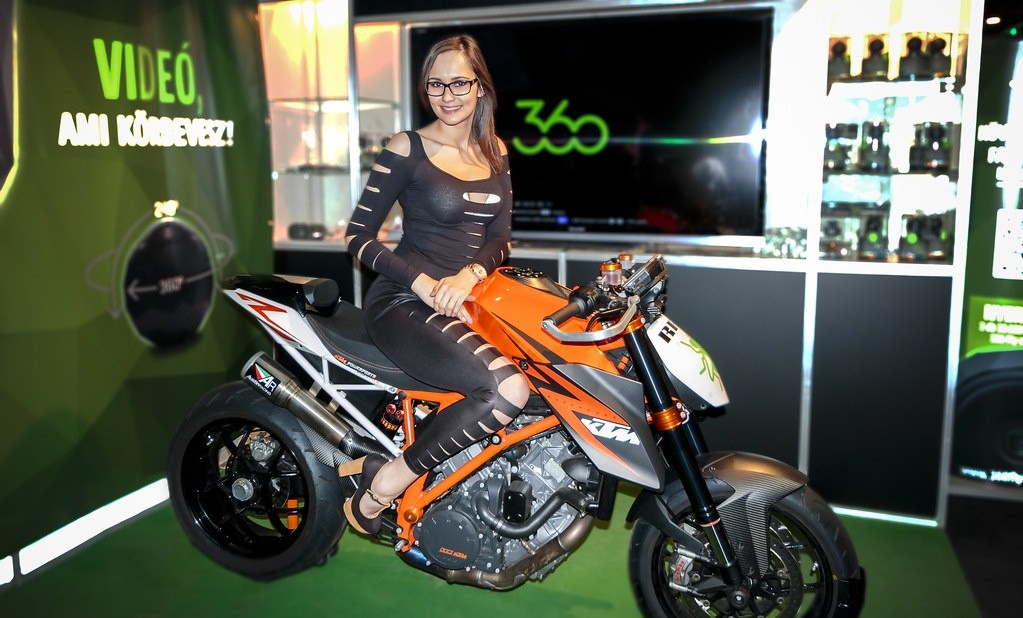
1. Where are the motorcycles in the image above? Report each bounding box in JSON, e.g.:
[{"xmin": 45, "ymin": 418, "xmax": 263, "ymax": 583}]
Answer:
[{"xmin": 170, "ymin": 250, "xmax": 867, "ymax": 618}]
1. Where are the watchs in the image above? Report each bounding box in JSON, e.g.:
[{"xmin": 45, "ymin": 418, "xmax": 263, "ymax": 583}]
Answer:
[{"xmin": 461, "ymin": 263, "xmax": 488, "ymax": 284}]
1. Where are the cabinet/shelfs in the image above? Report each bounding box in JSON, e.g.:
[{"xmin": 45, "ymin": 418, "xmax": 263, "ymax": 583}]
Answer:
[{"xmin": 273, "ymin": 1, "xmax": 988, "ymax": 530}]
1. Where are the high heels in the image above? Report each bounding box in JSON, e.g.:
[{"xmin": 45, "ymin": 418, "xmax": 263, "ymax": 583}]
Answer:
[{"xmin": 341, "ymin": 452, "xmax": 390, "ymax": 536}]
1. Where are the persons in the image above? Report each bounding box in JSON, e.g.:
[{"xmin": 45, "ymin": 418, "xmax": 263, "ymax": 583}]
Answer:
[{"xmin": 336, "ymin": 31, "xmax": 531, "ymax": 537}]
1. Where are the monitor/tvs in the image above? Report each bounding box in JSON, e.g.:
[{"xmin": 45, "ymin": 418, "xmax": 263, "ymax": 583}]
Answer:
[{"xmin": 403, "ymin": 5, "xmax": 777, "ymax": 235}]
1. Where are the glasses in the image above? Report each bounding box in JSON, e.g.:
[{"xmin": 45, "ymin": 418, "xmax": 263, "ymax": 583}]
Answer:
[{"xmin": 426, "ymin": 78, "xmax": 478, "ymax": 97}]
